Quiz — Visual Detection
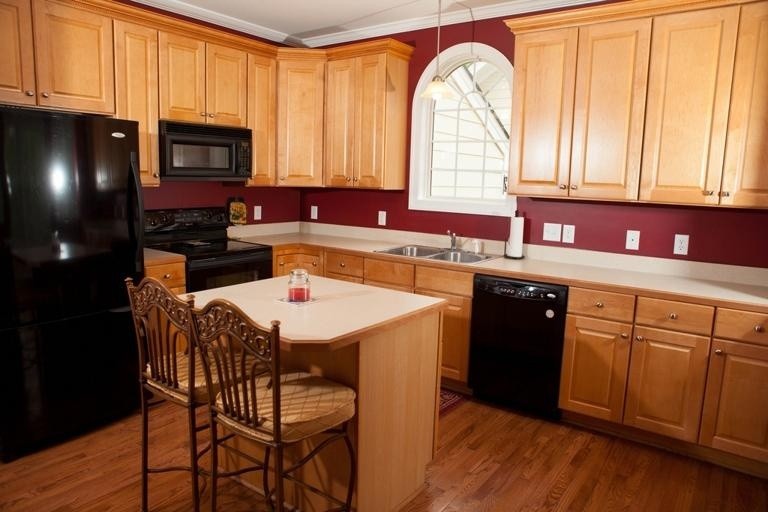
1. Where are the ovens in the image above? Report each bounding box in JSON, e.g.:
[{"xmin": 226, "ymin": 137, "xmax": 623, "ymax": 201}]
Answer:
[{"xmin": 185, "ymin": 250, "xmax": 274, "ymax": 292}]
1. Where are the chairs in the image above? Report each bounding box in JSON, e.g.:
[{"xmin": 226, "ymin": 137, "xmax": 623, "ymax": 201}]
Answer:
[
  {"xmin": 123, "ymin": 273, "xmax": 275, "ymax": 512},
  {"xmin": 184, "ymin": 292, "xmax": 359, "ymax": 511}
]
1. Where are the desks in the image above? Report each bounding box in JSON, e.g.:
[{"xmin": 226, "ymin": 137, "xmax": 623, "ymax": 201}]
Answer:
[{"xmin": 174, "ymin": 266, "xmax": 452, "ymax": 511}]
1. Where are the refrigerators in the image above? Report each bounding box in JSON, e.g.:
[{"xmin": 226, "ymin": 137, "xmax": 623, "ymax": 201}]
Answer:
[{"xmin": 0, "ymin": 105, "xmax": 147, "ymax": 463}]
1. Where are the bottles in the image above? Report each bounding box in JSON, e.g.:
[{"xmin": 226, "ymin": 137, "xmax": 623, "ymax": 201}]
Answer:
[{"xmin": 288, "ymin": 268, "xmax": 310, "ymax": 302}]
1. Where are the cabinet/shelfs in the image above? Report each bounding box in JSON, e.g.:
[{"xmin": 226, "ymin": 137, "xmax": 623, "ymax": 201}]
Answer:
[
  {"xmin": 273, "ymin": 243, "xmax": 323, "ymax": 284},
  {"xmin": 697, "ymin": 297, "xmax": 767, "ymax": 478},
  {"xmin": 326, "ymin": 38, "xmax": 413, "ymax": 190},
  {"xmin": 558, "ymin": 282, "xmax": 716, "ymax": 456},
  {"xmin": 247, "ymin": 31, "xmax": 277, "ymax": 188},
  {"xmin": 0, "ymin": 0, "xmax": 115, "ymax": 115},
  {"xmin": 638, "ymin": 0, "xmax": 768, "ymax": 208},
  {"xmin": 502, "ymin": 2, "xmax": 652, "ymax": 199},
  {"xmin": 277, "ymin": 49, "xmax": 326, "ymax": 189},
  {"xmin": 324, "ymin": 247, "xmax": 364, "ymax": 284},
  {"xmin": 158, "ymin": 30, "xmax": 247, "ymax": 129},
  {"xmin": 113, "ymin": 21, "xmax": 158, "ymax": 184},
  {"xmin": 144, "ymin": 250, "xmax": 187, "ymax": 405},
  {"xmin": 363, "ymin": 255, "xmax": 474, "ymax": 386}
]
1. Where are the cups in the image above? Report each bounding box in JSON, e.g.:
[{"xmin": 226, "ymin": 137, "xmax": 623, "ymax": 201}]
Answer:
[{"xmin": 472, "ymin": 238, "xmax": 482, "ymax": 254}]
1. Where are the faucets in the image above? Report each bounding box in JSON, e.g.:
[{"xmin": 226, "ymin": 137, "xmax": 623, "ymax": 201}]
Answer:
[{"xmin": 446, "ymin": 229, "xmax": 458, "ymax": 250}]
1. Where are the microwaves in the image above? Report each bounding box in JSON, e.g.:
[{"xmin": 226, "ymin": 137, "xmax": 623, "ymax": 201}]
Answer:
[{"xmin": 157, "ymin": 119, "xmax": 252, "ymax": 181}]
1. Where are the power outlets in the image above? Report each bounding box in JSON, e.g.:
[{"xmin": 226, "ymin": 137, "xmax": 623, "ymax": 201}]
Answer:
[{"xmin": 672, "ymin": 234, "xmax": 690, "ymax": 255}]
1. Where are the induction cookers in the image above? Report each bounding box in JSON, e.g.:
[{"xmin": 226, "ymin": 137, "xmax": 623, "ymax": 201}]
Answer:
[{"xmin": 144, "ymin": 239, "xmax": 273, "ymax": 260}]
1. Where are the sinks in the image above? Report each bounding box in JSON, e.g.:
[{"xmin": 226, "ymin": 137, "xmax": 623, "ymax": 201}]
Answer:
[
  {"xmin": 426, "ymin": 249, "xmax": 504, "ymax": 266},
  {"xmin": 376, "ymin": 243, "xmax": 449, "ymax": 258}
]
